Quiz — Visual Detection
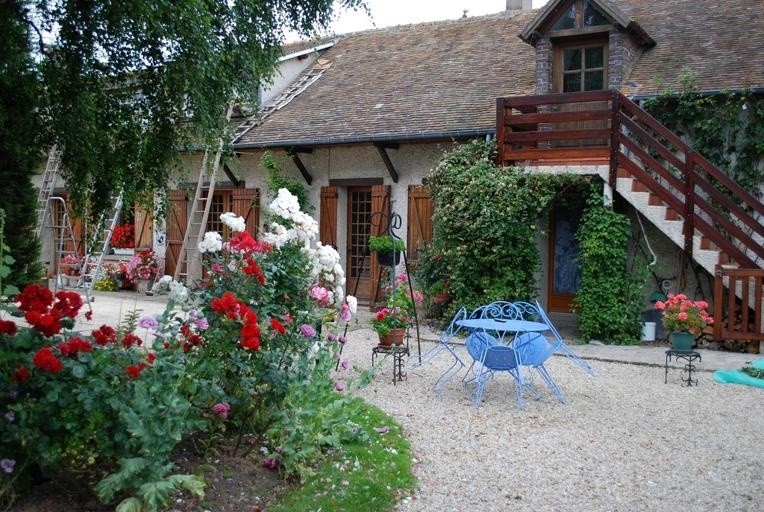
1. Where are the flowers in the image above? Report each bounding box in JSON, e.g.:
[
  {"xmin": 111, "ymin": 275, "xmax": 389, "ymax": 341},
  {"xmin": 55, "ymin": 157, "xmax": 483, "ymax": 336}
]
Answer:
[
  {"xmin": 62, "ymin": 253, "xmax": 83, "ymax": 276},
  {"xmin": 366, "ymin": 306, "xmax": 411, "ymax": 339},
  {"xmin": 655, "ymin": 293, "xmax": 715, "ymax": 334},
  {"xmin": 126, "ymin": 248, "xmax": 163, "ymax": 284},
  {"xmin": 109, "ymin": 224, "xmax": 135, "ymax": 249}
]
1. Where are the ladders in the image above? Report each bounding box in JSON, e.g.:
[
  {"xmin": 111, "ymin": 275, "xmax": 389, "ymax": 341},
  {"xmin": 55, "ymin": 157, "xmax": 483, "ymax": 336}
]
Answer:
[
  {"xmin": 32, "ymin": 137, "xmax": 66, "ymax": 239},
  {"xmin": 173, "ymin": 97, "xmax": 236, "ymax": 286},
  {"xmin": 74, "ymin": 127, "xmax": 148, "ymax": 298},
  {"xmin": 46, "ymin": 197, "xmax": 94, "ymax": 316}
]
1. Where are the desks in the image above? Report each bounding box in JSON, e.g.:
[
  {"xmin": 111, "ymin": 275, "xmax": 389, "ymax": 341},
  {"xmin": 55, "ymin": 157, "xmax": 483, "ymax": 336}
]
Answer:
[
  {"xmin": 664, "ymin": 351, "xmax": 701, "ymax": 387},
  {"xmin": 371, "ymin": 345, "xmax": 410, "ymax": 384}
]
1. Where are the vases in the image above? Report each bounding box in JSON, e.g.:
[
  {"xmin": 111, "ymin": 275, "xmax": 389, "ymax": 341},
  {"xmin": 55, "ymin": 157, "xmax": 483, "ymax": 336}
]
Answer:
[
  {"xmin": 111, "ymin": 248, "xmax": 134, "ymax": 255},
  {"xmin": 134, "ymin": 279, "xmax": 154, "ymax": 293},
  {"xmin": 57, "ymin": 274, "xmax": 69, "ymax": 285},
  {"xmin": 69, "ymin": 276, "xmax": 81, "ymax": 287},
  {"xmin": 670, "ymin": 332, "xmax": 696, "ymax": 353},
  {"xmin": 378, "ymin": 328, "xmax": 405, "ymax": 346}
]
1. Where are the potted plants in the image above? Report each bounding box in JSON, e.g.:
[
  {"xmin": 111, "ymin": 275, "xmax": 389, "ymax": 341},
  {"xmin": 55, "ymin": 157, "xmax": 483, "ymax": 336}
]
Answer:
[{"xmin": 369, "ymin": 235, "xmax": 406, "ymax": 267}]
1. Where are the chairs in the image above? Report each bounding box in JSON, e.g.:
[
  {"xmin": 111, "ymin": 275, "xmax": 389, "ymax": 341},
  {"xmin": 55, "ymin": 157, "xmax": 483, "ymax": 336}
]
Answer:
[{"xmin": 406, "ymin": 301, "xmax": 597, "ymax": 410}]
